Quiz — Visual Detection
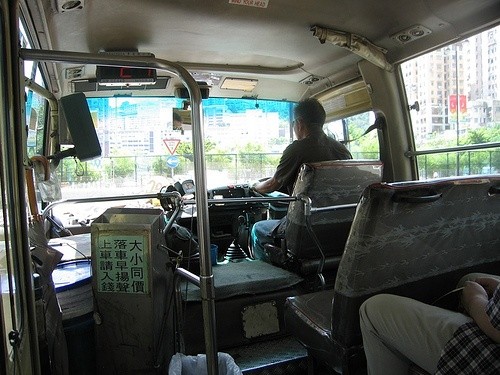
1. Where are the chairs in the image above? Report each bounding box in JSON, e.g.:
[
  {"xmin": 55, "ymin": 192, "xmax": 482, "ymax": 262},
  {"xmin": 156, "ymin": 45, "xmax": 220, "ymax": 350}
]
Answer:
[{"xmin": 265, "ymin": 158, "xmax": 383, "ymax": 275}]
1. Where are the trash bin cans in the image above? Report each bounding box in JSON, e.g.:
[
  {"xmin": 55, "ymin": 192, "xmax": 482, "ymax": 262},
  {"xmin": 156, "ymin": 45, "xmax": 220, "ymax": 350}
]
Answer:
[{"xmin": 169, "ymin": 354, "xmax": 242, "ymax": 374}]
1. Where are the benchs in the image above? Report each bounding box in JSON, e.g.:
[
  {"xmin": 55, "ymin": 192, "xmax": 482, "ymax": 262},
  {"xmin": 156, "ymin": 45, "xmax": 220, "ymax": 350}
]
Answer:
[{"xmin": 283, "ymin": 175, "xmax": 500, "ymax": 375}]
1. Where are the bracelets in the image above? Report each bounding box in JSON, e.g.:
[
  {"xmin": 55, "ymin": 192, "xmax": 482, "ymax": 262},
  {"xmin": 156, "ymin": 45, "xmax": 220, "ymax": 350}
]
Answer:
[{"xmin": 252, "ymin": 182, "xmax": 260, "ymax": 192}]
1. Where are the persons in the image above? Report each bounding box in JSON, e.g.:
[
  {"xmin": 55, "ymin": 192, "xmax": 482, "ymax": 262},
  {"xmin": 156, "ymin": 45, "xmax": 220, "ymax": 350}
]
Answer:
[
  {"xmin": 358, "ymin": 272, "xmax": 500, "ymax": 375},
  {"xmin": 248, "ymin": 99, "xmax": 361, "ymax": 264}
]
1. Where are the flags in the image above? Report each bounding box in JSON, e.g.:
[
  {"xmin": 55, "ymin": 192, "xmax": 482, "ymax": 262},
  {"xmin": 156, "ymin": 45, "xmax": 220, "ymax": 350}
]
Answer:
[
  {"xmin": 449, "ymin": 94, "xmax": 457, "ymax": 113},
  {"xmin": 460, "ymin": 94, "xmax": 466, "ymax": 113}
]
